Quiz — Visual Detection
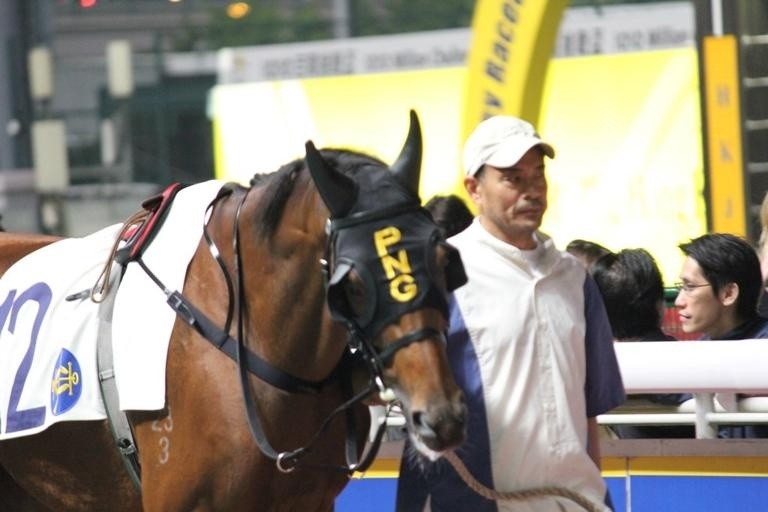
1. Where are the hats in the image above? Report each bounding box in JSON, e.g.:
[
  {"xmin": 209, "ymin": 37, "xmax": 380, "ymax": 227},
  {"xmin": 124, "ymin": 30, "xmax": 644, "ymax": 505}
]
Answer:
[{"xmin": 464, "ymin": 113, "xmax": 556, "ymax": 176}]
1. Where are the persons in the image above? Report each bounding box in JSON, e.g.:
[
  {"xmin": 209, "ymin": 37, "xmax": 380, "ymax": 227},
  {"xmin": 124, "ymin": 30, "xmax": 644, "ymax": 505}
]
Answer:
[
  {"xmin": 564, "ymin": 237, "xmax": 612, "ymax": 273},
  {"xmin": 669, "ymin": 233, "xmax": 766, "ymax": 439},
  {"xmin": 424, "ymin": 194, "xmax": 473, "ymax": 242},
  {"xmin": 353, "ymin": 114, "xmax": 626, "ymax": 512},
  {"xmin": 589, "ymin": 249, "xmax": 694, "ymax": 439}
]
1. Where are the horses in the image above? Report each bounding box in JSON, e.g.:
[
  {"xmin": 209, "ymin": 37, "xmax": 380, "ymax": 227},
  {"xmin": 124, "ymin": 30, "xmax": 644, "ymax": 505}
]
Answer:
[{"xmin": 1, "ymin": 108, "xmax": 472, "ymax": 512}]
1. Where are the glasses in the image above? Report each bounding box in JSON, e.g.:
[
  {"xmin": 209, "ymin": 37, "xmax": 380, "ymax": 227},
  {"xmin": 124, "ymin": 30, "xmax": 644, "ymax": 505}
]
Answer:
[{"xmin": 674, "ymin": 279, "xmax": 711, "ymax": 291}]
